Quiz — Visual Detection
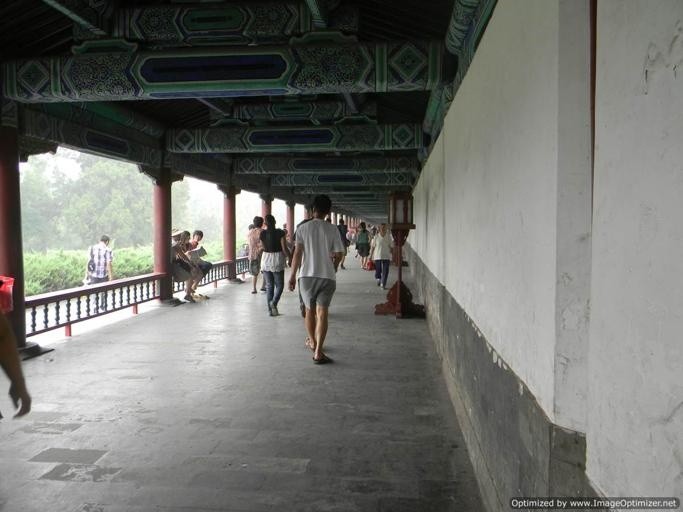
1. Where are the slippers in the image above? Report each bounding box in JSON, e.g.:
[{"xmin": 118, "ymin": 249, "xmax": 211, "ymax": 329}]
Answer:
[
  {"xmin": 305, "ymin": 338, "xmax": 330, "ymax": 364},
  {"xmin": 252, "ymin": 288, "xmax": 266, "ymax": 293}
]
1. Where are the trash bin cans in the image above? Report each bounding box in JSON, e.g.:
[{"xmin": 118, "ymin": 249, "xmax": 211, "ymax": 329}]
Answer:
[{"xmin": 240, "ymin": 244, "xmax": 249, "ymax": 257}]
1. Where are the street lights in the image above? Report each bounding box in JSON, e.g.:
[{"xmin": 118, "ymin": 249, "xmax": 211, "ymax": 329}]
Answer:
[{"xmin": 375, "ymin": 191, "xmax": 425, "ymax": 318}]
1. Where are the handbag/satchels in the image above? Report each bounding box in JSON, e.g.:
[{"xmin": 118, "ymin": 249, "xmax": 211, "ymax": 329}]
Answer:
[
  {"xmin": 88, "ymin": 259, "xmax": 95, "ymax": 271},
  {"xmin": 367, "ymin": 260, "xmax": 375, "ymax": 270}
]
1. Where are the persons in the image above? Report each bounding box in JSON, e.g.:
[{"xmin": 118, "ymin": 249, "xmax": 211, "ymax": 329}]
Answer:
[
  {"xmin": 0, "ymin": 275, "xmax": 32, "ymax": 419},
  {"xmin": 288, "ymin": 194, "xmax": 346, "ymax": 363},
  {"xmin": 246, "ymin": 201, "xmax": 317, "ymax": 318},
  {"xmin": 170, "ymin": 229, "xmax": 213, "ymax": 302},
  {"xmin": 326, "ymin": 216, "xmax": 394, "ymax": 290},
  {"xmin": 85, "ymin": 234, "xmax": 114, "ymax": 314}
]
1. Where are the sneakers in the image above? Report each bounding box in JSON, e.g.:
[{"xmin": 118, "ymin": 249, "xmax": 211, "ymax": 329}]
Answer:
[
  {"xmin": 184, "ymin": 288, "xmax": 196, "ymax": 303},
  {"xmin": 377, "ymin": 278, "xmax": 385, "ymax": 290},
  {"xmin": 268, "ymin": 300, "xmax": 278, "ymax": 316}
]
